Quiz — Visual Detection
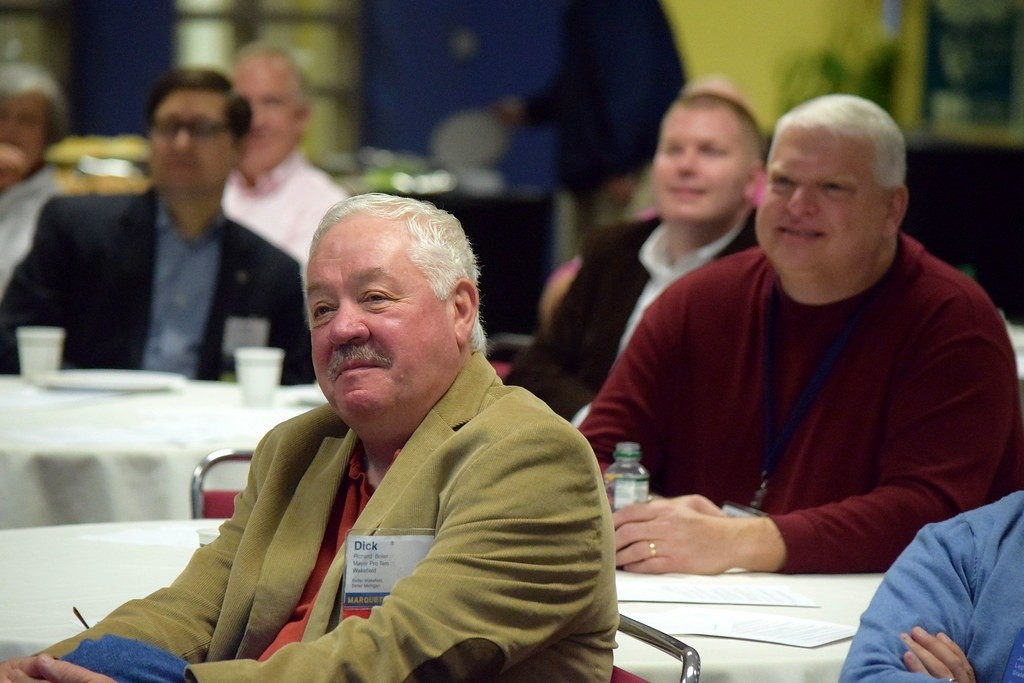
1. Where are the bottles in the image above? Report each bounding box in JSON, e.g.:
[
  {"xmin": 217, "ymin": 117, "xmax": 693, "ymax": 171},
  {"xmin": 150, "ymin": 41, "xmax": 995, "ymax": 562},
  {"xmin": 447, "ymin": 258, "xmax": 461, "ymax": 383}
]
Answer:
[{"xmin": 602, "ymin": 442, "xmax": 650, "ymax": 513}]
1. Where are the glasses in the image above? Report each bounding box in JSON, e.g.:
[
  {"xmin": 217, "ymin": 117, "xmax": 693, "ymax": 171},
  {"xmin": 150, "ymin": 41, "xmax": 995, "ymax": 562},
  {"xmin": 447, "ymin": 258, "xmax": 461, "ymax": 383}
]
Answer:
[{"xmin": 149, "ymin": 113, "xmax": 229, "ymax": 137}]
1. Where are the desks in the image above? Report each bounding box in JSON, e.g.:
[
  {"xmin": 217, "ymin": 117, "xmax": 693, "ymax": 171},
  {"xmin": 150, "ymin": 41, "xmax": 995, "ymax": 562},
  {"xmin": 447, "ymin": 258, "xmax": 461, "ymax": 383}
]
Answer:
[
  {"xmin": 0, "ymin": 514, "xmax": 897, "ymax": 681},
  {"xmin": 0, "ymin": 370, "xmax": 363, "ymax": 513}
]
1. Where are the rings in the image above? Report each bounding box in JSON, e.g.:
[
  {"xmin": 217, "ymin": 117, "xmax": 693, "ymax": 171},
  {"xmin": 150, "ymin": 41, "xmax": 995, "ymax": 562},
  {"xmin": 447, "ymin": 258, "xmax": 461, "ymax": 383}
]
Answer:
[
  {"xmin": 949, "ymin": 676, "xmax": 957, "ymax": 683},
  {"xmin": 647, "ymin": 540, "xmax": 657, "ymax": 558}
]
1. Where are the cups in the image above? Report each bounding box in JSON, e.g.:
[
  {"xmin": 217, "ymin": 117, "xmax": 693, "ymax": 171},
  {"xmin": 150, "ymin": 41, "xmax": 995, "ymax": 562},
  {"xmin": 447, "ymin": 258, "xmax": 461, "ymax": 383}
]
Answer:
[
  {"xmin": 16, "ymin": 326, "xmax": 66, "ymax": 382},
  {"xmin": 235, "ymin": 346, "xmax": 285, "ymax": 401}
]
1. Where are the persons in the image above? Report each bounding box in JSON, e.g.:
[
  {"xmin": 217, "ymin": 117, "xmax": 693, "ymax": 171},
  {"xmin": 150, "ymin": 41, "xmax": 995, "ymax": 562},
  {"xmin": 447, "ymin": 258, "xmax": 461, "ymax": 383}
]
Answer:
[
  {"xmin": 540, "ymin": 70, "xmax": 774, "ymax": 324},
  {"xmin": 499, "ymin": 90, "xmax": 770, "ymax": 432},
  {"xmin": 218, "ymin": 41, "xmax": 354, "ymax": 284},
  {"xmin": 1, "ymin": 64, "xmax": 317, "ymax": 390},
  {"xmin": 834, "ymin": 489, "xmax": 1023, "ymax": 683},
  {"xmin": 491, "ymin": 0, "xmax": 689, "ymax": 269},
  {"xmin": 0, "ymin": 190, "xmax": 619, "ymax": 682},
  {"xmin": 0, "ymin": 57, "xmax": 71, "ymax": 304},
  {"xmin": 575, "ymin": 84, "xmax": 1024, "ymax": 579}
]
